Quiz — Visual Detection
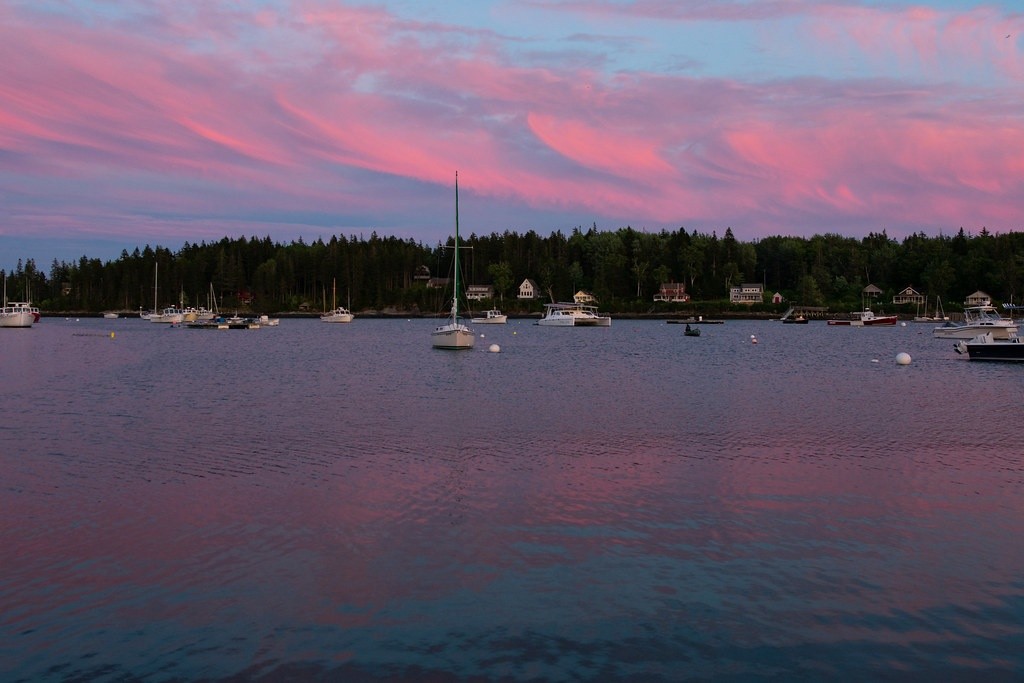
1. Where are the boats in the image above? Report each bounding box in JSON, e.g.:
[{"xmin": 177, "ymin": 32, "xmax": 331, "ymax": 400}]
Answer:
[
  {"xmin": 532, "ymin": 302, "xmax": 612, "ymax": 327},
  {"xmin": 911, "ymin": 295, "xmax": 950, "ymax": 322},
  {"xmin": 104, "ymin": 313, "xmax": 119, "ymax": 319},
  {"xmin": 471, "ymin": 307, "xmax": 508, "ymax": 324},
  {"xmin": 139, "ymin": 262, "xmax": 280, "ymax": 329},
  {"xmin": 965, "ymin": 331, "xmax": 1024, "ymax": 360},
  {"xmin": 782, "ymin": 308, "xmax": 809, "ymax": 324},
  {"xmin": 933, "ymin": 318, "xmax": 1020, "ymax": 339},
  {"xmin": 780, "ymin": 305, "xmax": 841, "ymax": 320},
  {"xmin": 667, "ymin": 316, "xmax": 725, "ymax": 324},
  {"xmin": 828, "ymin": 307, "xmax": 898, "ymax": 325},
  {"xmin": 0, "ymin": 276, "xmax": 41, "ymax": 327},
  {"xmin": 684, "ymin": 323, "xmax": 701, "ymax": 336},
  {"xmin": 319, "ymin": 277, "xmax": 354, "ymax": 322}
]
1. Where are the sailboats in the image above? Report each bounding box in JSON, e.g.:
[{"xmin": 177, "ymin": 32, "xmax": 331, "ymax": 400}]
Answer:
[{"xmin": 430, "ymin": 170, "xmax": 476, "ymax": 349}]
